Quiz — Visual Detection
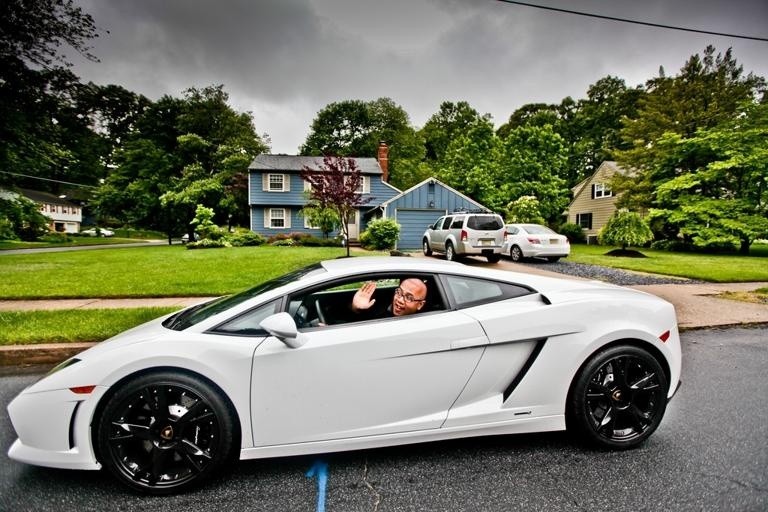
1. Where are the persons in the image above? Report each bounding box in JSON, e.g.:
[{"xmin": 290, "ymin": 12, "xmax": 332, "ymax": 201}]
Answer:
[{"xmin": 318, "ymin": 277, "xmax": 429, "ymax": 328}]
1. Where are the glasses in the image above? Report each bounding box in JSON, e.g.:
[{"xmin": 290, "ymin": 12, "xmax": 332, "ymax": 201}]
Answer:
[{"xmin": 394, "ymin": 287, "xmax": 424, "ymax": 303}]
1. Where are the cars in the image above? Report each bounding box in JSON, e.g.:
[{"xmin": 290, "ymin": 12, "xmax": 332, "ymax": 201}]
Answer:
[
  {"xmin": 8, "ymin": 256, "xmax": 682, "ymax": 495},
  {"xmin": 501, "ymin": 224, "xmax": 571, "ymax": 263},
  {"xmin": 182, "ymin": 233, "xmax": 201, "ymax": 243},
  {"xmin": 82, "ymin": 228, "xmax": 115, "ymax": 237}
]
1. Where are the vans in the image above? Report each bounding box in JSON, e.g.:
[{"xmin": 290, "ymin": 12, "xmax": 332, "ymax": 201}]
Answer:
[{"xmin": 423, "ymin": 207, "xmax": 509, "ymax": 263}]
